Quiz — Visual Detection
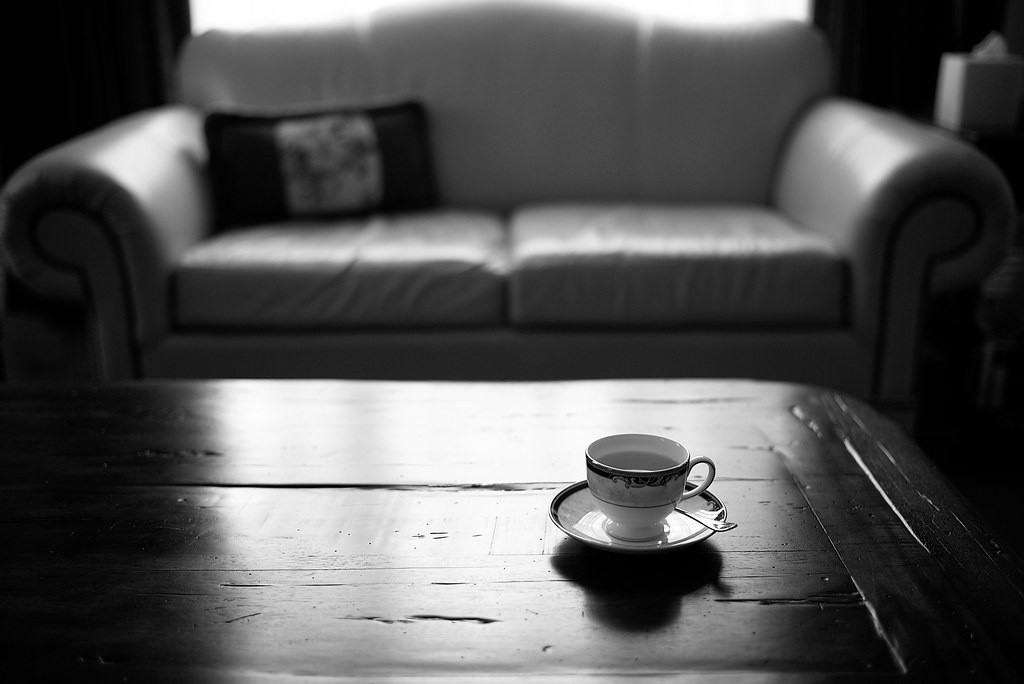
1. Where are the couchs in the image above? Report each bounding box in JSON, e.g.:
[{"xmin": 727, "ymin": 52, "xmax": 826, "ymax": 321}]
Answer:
[{"xmin": 2, "ymin": 0, "xmax": 1024, "ymax": 441}]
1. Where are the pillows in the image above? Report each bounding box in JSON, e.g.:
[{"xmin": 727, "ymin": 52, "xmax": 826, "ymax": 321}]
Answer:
[{"xmin": 202, "ymin": 100, "xmax": 441, "ymax": 234}]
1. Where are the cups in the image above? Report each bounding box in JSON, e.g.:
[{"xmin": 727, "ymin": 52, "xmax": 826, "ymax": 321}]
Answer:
[{"xmin": 586, "ymin": 434, "xmax": 716, "ymax": 541}]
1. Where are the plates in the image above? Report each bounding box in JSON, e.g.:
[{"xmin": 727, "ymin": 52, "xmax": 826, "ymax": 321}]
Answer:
[{"xmin": 547, "ymin": 481, "xmax": 727, "ymax": 552}]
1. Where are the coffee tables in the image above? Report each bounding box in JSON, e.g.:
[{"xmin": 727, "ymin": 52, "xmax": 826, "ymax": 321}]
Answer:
[{"xmin": 0, "ymin": 378, "xmax": 1024, "ymax": 684}]
